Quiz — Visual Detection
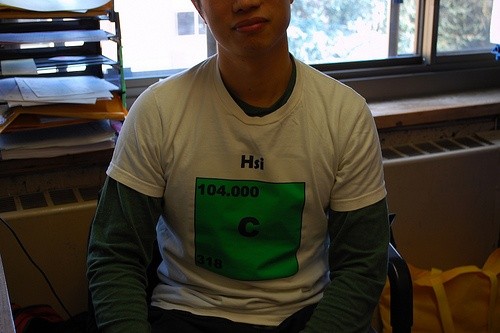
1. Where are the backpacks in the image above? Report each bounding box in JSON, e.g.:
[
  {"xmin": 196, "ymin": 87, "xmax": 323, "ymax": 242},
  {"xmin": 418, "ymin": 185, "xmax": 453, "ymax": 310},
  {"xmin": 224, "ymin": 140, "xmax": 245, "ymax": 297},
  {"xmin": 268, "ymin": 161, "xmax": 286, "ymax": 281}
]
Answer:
[{"xmin": 12, "ymin": 302, "xmax": 65, "ymax": 333}]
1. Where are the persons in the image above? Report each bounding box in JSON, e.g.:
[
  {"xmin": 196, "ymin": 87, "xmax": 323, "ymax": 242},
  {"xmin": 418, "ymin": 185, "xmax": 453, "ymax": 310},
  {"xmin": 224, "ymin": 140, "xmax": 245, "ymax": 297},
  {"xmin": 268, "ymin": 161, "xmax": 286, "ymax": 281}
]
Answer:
[{"xmin": 83, "ymin": 0, "xmax": 391, "ymax": 333}]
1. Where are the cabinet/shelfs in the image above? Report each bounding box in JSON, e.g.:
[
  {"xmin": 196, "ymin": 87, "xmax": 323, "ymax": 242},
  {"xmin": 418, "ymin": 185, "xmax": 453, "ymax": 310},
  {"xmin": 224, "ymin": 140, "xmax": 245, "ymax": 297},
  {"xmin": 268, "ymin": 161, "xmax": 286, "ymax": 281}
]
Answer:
[{"xmin": 0, "ymin": 0, "xmax": 127, "ymax": 132}]
